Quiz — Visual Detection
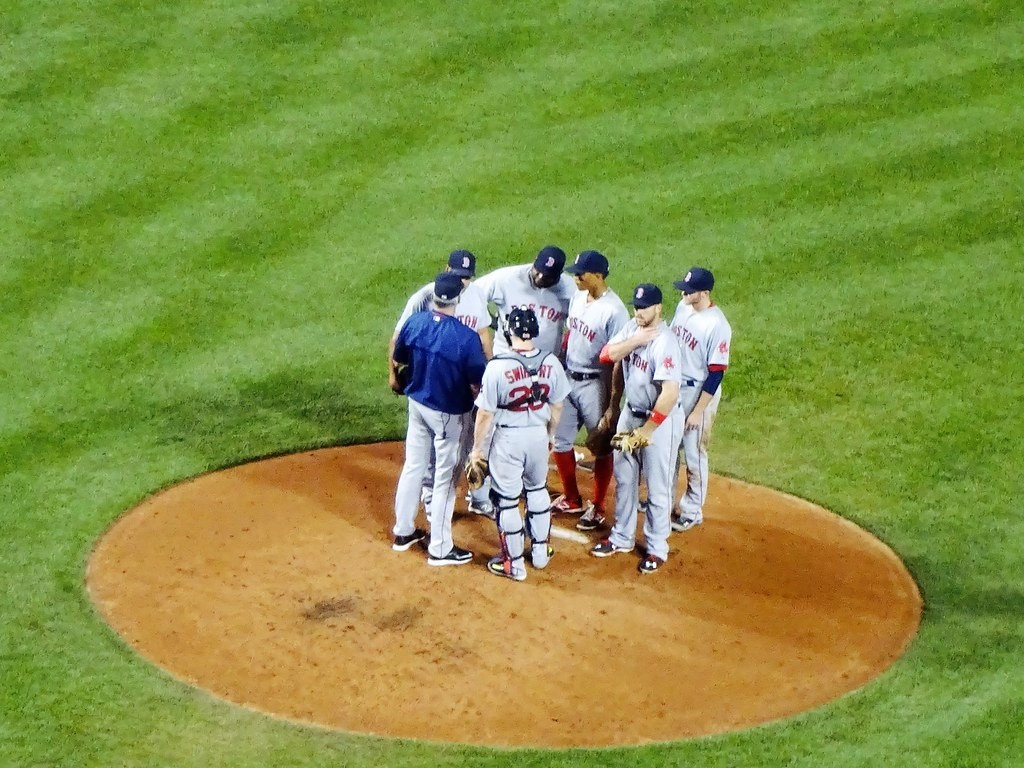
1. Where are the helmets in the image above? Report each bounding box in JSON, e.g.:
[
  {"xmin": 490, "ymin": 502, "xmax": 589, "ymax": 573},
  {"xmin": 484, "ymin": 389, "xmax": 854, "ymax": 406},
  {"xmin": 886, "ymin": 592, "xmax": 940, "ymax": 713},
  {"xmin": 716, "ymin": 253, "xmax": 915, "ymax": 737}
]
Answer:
[{"xmin": 509, "ymin": 307, "xmax": 539, "ymax": 340}]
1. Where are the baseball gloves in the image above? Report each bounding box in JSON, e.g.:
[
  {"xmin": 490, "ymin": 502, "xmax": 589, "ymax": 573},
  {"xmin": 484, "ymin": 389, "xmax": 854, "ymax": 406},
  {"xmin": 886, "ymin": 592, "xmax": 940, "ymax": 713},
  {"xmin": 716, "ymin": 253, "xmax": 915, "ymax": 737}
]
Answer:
[
  {"xmin": 463, "ymin": 456, "xmax": 490, "ymax": 490},
  {"xmin": 585, "ymin": 413, "xmax": 617, "ymax": 458},
  {"xmin": 608, "ymin": 426, "xmax": 653, "ymax": 454}
]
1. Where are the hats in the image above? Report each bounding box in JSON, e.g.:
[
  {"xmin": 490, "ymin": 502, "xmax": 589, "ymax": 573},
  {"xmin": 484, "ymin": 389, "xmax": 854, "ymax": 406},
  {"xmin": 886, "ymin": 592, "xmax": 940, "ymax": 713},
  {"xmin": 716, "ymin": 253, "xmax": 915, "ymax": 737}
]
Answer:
[
  {"xmin": 449, "ymin": 250, "xmax": 476, "ymax": 279},
  {"xmin": 627, "ymin": 283, "xmax": 663, "ymax": 309},
  {"xmin": 674, "ymin": 267, "xmax": 715, "ymax": 294},
  {"xmin": 535, "ymin": 246, "xmax": 566, "ymax": 277},
  {"xmin": 435, "ymin": 272, "xmax": 465, "ymax": 304},
  {"xmin": 565, "ymin": 250, "xmax": 610, "ymax": 274}
]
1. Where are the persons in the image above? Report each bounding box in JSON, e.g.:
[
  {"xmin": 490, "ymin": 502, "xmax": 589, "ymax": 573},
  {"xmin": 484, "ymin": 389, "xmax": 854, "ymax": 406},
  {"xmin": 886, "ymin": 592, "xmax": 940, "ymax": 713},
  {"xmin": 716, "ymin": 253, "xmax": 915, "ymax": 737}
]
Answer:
[
  {"xmin": 388, "ymin": 250, "xmax": 495, "ymax": 522},
  {"xmin": 464, "ymin": 308, "xmax": 571, "ymax": 580},
  {"xmin": 638, "ymin": 267, "xmax": 731, "ymax": 531},
  {"xmin": 550, "ymin": 249, "xmax": 630, "ymax": 528},
  {"xmin": 591, "ymin": 284, "xmax": 686, "ymax": 572},
  {"xmin": 393, "ymin": 272, "xmax": 486, "ymax": 565},
  {"xmin": 474, "ymin": 246, "xmax": 578, "ymax": 499}
]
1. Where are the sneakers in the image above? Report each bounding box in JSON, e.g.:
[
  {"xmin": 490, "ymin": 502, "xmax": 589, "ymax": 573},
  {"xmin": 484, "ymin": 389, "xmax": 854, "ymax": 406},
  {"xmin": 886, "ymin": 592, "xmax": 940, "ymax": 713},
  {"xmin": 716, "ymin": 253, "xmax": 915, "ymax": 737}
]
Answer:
[
  {"xmin": 576, "ymin": 499, "xmax": 606, "ymax": 530},
  {"xmin": 468, "ymin": 498, "xmax": 497, "ymax": 519},
  {"xmin": 423, "ymin": 496, "xmax": 432, "ymax": 523},
  {"xmin": 639, "ymin": 555, "xmax": 664, "ymax": 574},
  {"xmin": 580, "ymin": 459, "xmax": 595, "ymax": 471},
  {"xmin": 392, "ymin": 528, "xmax": 425, "ymax": 550},
  {"xmin": 670, "ymin": 516, "xmax": 703, "ymax": 532},
  {"xmin": 637, "ymin": 500, "xmax": 649, "ymax": 512},
  {"xmin": 487, "ymin": 557, "xmax": 521, "ymax": 582},
  {"xmin": 551, "ymin": 494, "xmax": 585, "ymax": 514},
  {"xmin": 428, "ymin": 544, "xmax": 474, "ymax": 566},
  {"xmin": 590, "ymin": 539, "xmax": 635, "ymax": 556},
  {"xmin": 543, "ymin": 545, "xmax": 554, "ymax": 570}
]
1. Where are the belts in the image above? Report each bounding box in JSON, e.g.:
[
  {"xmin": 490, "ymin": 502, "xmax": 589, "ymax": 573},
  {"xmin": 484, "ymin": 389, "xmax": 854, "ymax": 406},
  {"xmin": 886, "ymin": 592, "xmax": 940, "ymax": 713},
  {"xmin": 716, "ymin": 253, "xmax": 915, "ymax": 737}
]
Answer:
[
  {"xmin": 686, "ymin": 379, "xmax": 695, "ymax": 387},
  {"xmin": 627, "ymin": 403, "xmax": 647, "ymax": 421},
  {"xmin": 501, "ymin": 424, "xmax": 508, "ymax": 428},
  {"xmin": 566, "ymin": 367, "xmax": 600, "ymax": 381}
]
